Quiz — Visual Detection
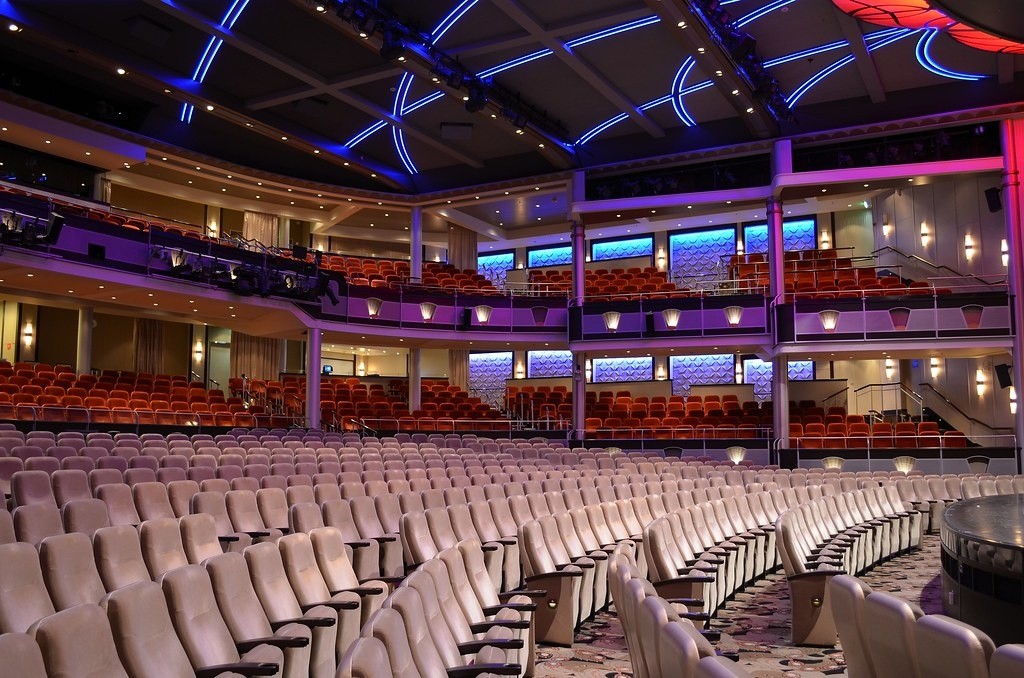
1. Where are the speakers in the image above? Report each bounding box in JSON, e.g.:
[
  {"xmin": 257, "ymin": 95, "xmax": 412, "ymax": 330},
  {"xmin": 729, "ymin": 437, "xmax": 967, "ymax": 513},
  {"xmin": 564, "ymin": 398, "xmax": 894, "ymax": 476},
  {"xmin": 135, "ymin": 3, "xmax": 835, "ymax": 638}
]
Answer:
[
  {"xmin": 568, "ymin": 305, "xmax": 583, "ymax": 341},
  {"xmin": 995, "ymin": 364, "xmax": 1012, "ymax": 389},
  {"xmin": 984, "ymin": 187, "xmax": 1003, "ymax": 213},
  {"xmin": 44, "ymin": 212, "xmax": 64, "ymax": 245},
  {"xmin": 775, "ymin": 303, "xmax": 795, "ymax": 342}
]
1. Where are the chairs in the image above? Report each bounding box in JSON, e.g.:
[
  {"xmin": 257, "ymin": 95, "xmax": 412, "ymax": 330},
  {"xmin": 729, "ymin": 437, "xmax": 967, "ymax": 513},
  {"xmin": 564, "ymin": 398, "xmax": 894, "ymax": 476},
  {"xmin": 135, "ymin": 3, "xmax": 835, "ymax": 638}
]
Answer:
[
  {"xmin": 730, "ymin": 248, "xmax": 950, "ymax": 303},
  {"xmin": 75, "ymin": 205, "xmax": 498, "ymax": 297},
  {"xmin": 0, "ymin": 356, "xmax": 1024, "ymax": 678},
  {"xmin": 528, "ymin": 266, "xmax": 707, "ymax": 299}
]
